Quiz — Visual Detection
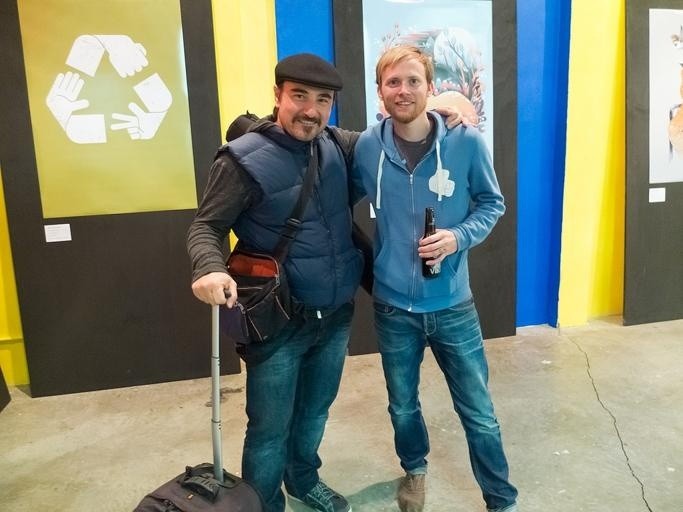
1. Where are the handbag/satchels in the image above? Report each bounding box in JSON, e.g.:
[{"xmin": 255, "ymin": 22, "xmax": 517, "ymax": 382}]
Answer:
[
  {"xmin": 220, "ymin": 251, "xmax": 293, "ymax": 345},
  {"xmin": 353, "ymin": 224, "xmax": 375, "ymax": 295}
]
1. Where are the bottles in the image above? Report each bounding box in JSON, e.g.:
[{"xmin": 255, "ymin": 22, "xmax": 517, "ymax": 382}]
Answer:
[{"xmin": 420, "ymin": 205, "xmax": 443, "ymax": 278}]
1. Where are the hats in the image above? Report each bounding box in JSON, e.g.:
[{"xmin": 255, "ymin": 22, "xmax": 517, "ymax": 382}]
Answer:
[{"xmin": 276, "ymin": 53, "xmax": 345, "ymax": 93}]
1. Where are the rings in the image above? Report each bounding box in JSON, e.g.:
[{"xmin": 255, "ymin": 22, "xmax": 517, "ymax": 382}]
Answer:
[{"xmin": 440, "ymin": 247, "xmax": 445, "ymax": 256}]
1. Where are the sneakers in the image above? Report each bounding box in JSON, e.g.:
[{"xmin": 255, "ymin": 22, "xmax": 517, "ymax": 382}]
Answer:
[
  {"xmin": 288, "ymin": 481, "xmax": 352, "ymax": 512},
  {"xmin": 398, "ymin": 473, "xmax": 425, "ymax": 512}
]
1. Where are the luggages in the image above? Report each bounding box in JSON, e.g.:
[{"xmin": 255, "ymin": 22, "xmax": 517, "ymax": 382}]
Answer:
[{"xmin": 133, "ymin": 290, "xmax": 263, "ymax": 512}]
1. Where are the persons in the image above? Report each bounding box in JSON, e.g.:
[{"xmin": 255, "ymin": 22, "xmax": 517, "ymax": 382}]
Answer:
[
  {"xmin": 320, "ymin": 44, "xmax": 521, "ymax": 511},
  {"xmin": 186, "ymin": 55, "xmax": 471, "ymax": 512}
]
